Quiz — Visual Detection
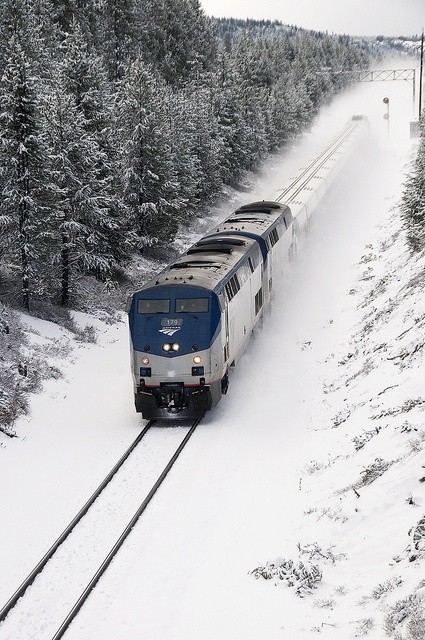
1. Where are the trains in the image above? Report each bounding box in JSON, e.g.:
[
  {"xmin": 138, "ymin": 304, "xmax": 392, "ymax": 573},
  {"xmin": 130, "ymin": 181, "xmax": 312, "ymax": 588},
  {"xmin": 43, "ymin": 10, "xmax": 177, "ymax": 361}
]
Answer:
[{"xmin": 127, "ymin": 113, "xmax": 370, "ymax": 422}]
